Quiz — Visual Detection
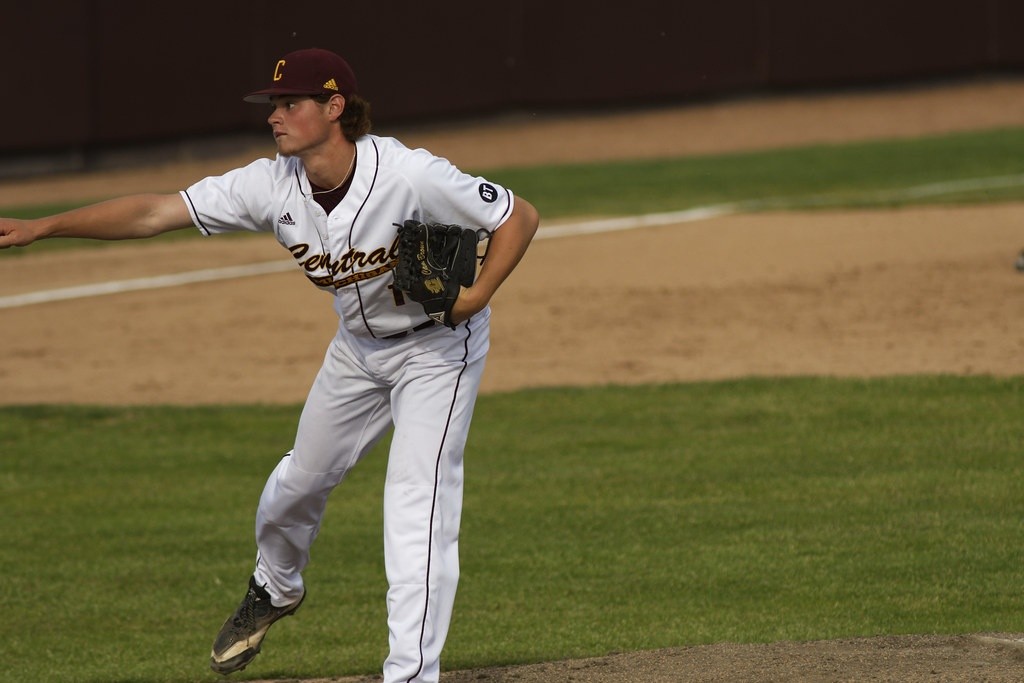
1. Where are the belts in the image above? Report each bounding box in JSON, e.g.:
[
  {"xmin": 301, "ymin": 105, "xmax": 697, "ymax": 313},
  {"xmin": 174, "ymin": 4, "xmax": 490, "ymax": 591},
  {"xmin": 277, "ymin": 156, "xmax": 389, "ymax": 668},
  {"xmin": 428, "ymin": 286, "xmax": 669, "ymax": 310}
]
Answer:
[{"xmin": 382, "ymin": 319, "xmax": 435, "ymax": 339}]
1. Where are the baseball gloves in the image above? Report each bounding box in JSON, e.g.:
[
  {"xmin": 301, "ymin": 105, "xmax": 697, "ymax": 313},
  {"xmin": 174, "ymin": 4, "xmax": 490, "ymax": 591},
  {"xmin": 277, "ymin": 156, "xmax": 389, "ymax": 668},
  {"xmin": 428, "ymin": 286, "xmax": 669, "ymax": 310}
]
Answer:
[{"xmin": 387, "ymin": 218, "xmax": 478, "ymax": 332}]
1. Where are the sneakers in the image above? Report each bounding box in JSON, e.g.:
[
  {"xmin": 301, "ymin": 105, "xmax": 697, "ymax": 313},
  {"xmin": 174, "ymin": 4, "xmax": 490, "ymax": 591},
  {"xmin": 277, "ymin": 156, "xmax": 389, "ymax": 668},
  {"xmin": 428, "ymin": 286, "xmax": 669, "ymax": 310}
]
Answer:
[{"xmin": 209, "ymin": 574, "xmax": 307, "ymax": 676}]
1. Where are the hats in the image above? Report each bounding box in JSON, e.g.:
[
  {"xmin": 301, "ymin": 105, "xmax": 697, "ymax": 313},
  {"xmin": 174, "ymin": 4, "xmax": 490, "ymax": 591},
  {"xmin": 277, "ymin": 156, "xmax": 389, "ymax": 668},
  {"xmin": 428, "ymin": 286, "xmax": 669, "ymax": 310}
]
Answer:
[{"xmin": 246, "ymin": 47, "xmax": 358, "ymax": 96}]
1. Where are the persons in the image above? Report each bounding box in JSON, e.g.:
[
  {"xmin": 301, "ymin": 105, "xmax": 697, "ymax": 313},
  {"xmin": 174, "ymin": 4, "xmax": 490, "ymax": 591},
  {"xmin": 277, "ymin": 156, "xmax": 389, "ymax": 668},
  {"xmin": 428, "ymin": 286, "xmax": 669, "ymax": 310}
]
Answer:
[{"xmin": 0, "ymin": 49, "xmax": 540, "ymax": 683}]
